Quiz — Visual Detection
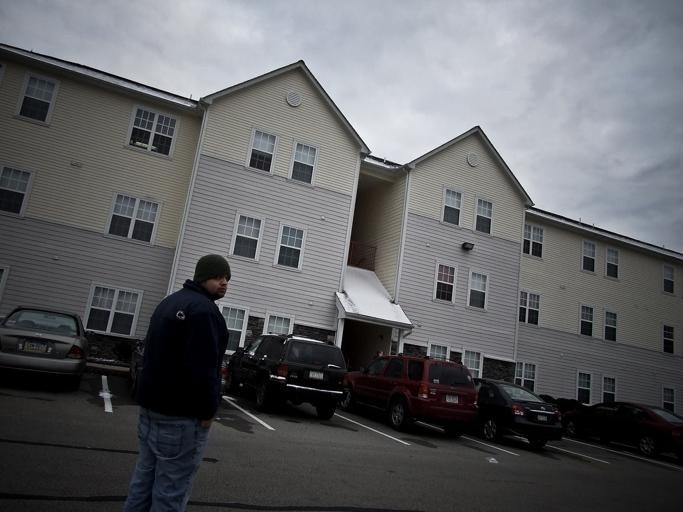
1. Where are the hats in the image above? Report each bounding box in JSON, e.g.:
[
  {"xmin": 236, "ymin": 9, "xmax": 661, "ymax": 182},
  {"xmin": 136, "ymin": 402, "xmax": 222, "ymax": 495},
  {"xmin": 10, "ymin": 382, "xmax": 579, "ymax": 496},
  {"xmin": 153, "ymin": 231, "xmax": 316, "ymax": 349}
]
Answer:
[{"xmin": 193, "ymin": 254, "xmax": 231, "ymax": 286}]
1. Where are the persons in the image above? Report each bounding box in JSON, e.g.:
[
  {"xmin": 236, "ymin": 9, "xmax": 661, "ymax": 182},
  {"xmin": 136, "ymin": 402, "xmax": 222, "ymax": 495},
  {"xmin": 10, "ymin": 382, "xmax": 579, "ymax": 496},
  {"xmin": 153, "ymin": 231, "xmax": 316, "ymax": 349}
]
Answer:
[
  {"xmin": 375, "ymin": 349, "xmax": 383, "ymax": 359},
  {"xmin": 120, "ymin": 255, "xmax": 232, "ymax": 512}
]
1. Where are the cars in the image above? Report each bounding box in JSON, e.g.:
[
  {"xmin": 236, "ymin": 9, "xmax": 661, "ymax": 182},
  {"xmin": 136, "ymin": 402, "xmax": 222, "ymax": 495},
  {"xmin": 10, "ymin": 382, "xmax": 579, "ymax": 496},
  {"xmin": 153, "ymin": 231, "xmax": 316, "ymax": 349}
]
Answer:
[{"xmin": 0, "ymin": 306, "xmax": 89, "ymax": 391}]
